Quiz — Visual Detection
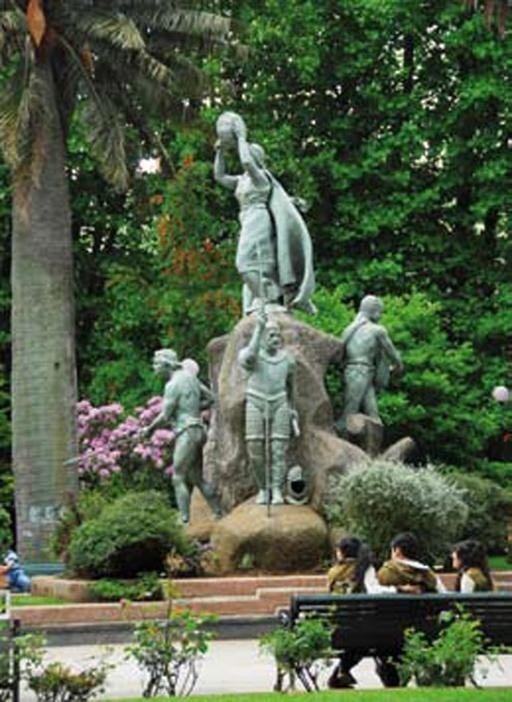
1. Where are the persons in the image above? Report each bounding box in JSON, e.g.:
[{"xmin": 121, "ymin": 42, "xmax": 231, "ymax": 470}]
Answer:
[
  {"xmin": 142, "ymin": 345, "xmax": 226, "ymax": 520},
  {"xmin": 325, "ymin": 531, "xmax": 497, "ymax": 688},
  {"xmin": 334, "ymin": 296, "xmax": 401, "ymax": 436},
  {"xmin": 0, "ymin": 541, "xmax": 31, "ymax": 592},
  {"xmin": 209, "ymin": 114, "xmax": 315, "ymax": 313},
  {"xmin": 238, "ymin": 302, "xmax": 305, "ymax": 505}
]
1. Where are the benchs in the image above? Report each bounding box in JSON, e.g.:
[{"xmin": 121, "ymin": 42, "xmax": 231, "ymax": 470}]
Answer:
[{"xmin": 273, "ymin": 591, "xmax": 512, "ymax": 691}]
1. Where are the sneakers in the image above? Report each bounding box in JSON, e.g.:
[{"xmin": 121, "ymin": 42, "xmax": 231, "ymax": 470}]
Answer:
[{"xmin": 326, "ymin": 667, "xmax": 357, "ymax": 687}]
